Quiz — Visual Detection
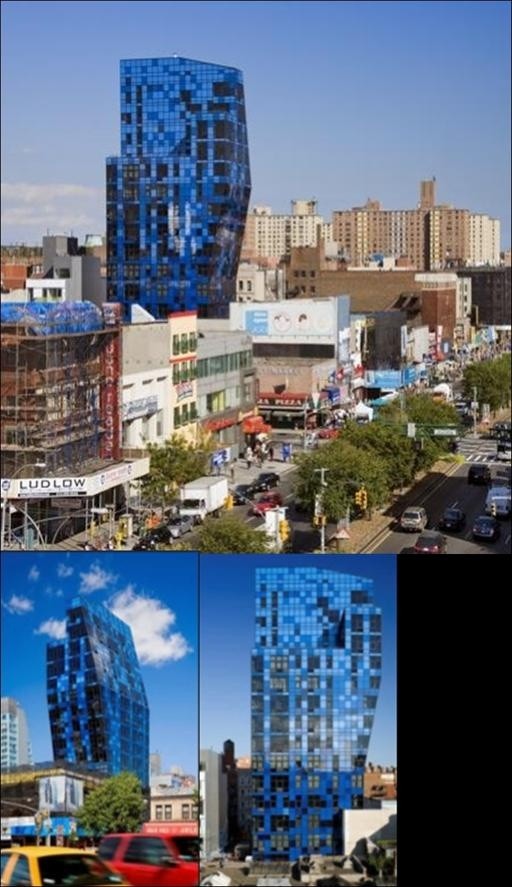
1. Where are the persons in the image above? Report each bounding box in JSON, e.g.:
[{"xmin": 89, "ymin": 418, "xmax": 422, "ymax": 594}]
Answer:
[{"xmin": 244, "ymin": 446, "xmax": 254, "ymax": 470}]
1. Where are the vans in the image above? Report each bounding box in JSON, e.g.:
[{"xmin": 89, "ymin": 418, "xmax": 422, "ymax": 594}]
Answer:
[{"xmin": 401, "ymin": 505, "xmax": 428, "ymax": 531}]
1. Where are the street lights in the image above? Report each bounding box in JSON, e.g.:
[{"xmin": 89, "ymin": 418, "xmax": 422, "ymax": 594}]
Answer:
[
  {"xmin": 0, "ymin": 460, "xmax": 47, "ymax": 552},
  {"xmin": 51, "ymin": 506, "xmax": 108, "ymax": 545}
]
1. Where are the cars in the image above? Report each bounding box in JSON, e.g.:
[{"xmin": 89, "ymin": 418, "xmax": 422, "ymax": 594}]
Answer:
[
  {"xmin": 438, "ymin": 505, "xmax": 466, "ymax": 532},
  {"xmin": 484, "ymin": 485, "xmax": 511, "ymax": 514},
  {"xmin": 253, "ymin": 490, "xmax": 281, "ymax": 516},
  {"xmin": 412, "ymin": 529, "xmax": 448, "ymax": 553},
  {"xmin": 472, "ymin": 514, "xmax": 500, "ymax": 542},
  {"xmin": 496, "ymin": 443, "xmax": 511, "ymax": 460},
  {"xmin": 230, "ymin": 484, "xmax": 254, "ymax": 505},
  {"xmin": 133, "ymin": 516, "xmax": 194, "ymax": 551},
  {"xmin": 97, "ymin": 830, "xmax": 198, "ymax": 886},
  {"xmin": 467, "ymin": 463, "xmax": 491, "ymax": 485},
  {"xmin": 2, "ymin": 845, "xmax": 131, "ymax": 887},
  {"xmin": 251, "ymin": 472, "xmax": 279, "ymax": 491}
]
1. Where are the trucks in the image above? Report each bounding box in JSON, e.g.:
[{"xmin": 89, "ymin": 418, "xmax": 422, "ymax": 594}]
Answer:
[{"xmin": 179, "ymin": 475, "xmax": 229, "ymax": 522}]
